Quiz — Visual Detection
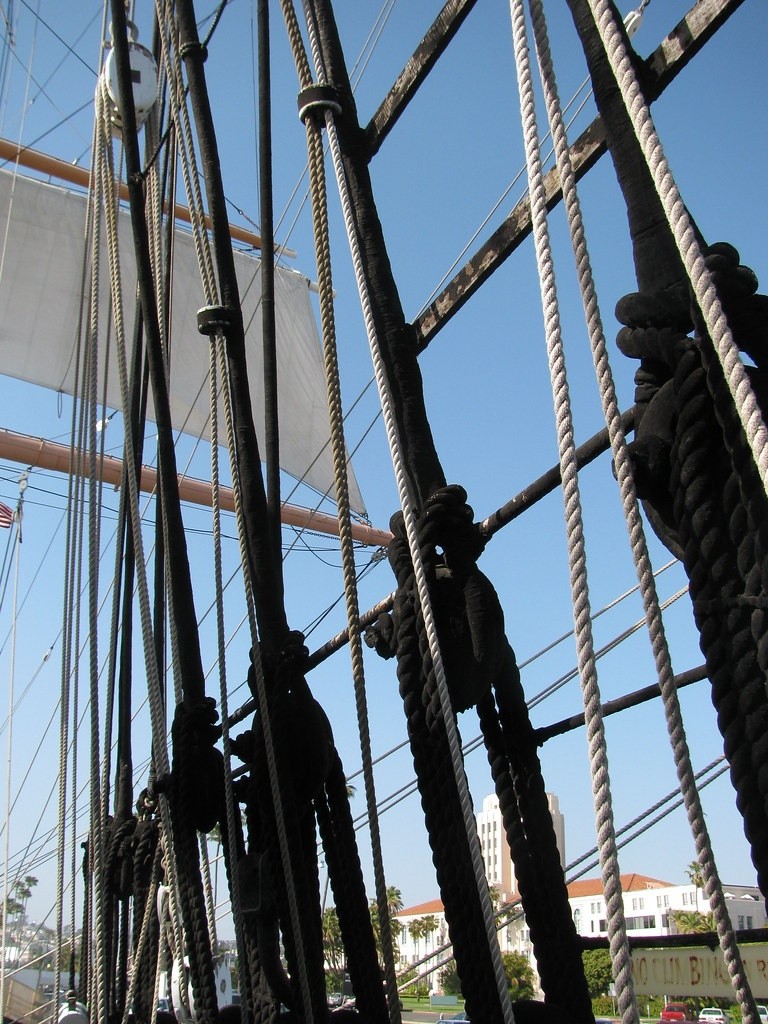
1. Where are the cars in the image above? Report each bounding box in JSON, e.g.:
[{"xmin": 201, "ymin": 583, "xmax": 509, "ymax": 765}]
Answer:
[
  {"xmin": 757, "ymin": 1004, "xmax": 768, "ymax": 1024},
  {"xmin": 659, "ymin": 1001, "xmax": 694, "ymax": 1022},
  {"xmin": 698, "ymin": 1007, "xmax": 731, "ymax": 1024}
]
